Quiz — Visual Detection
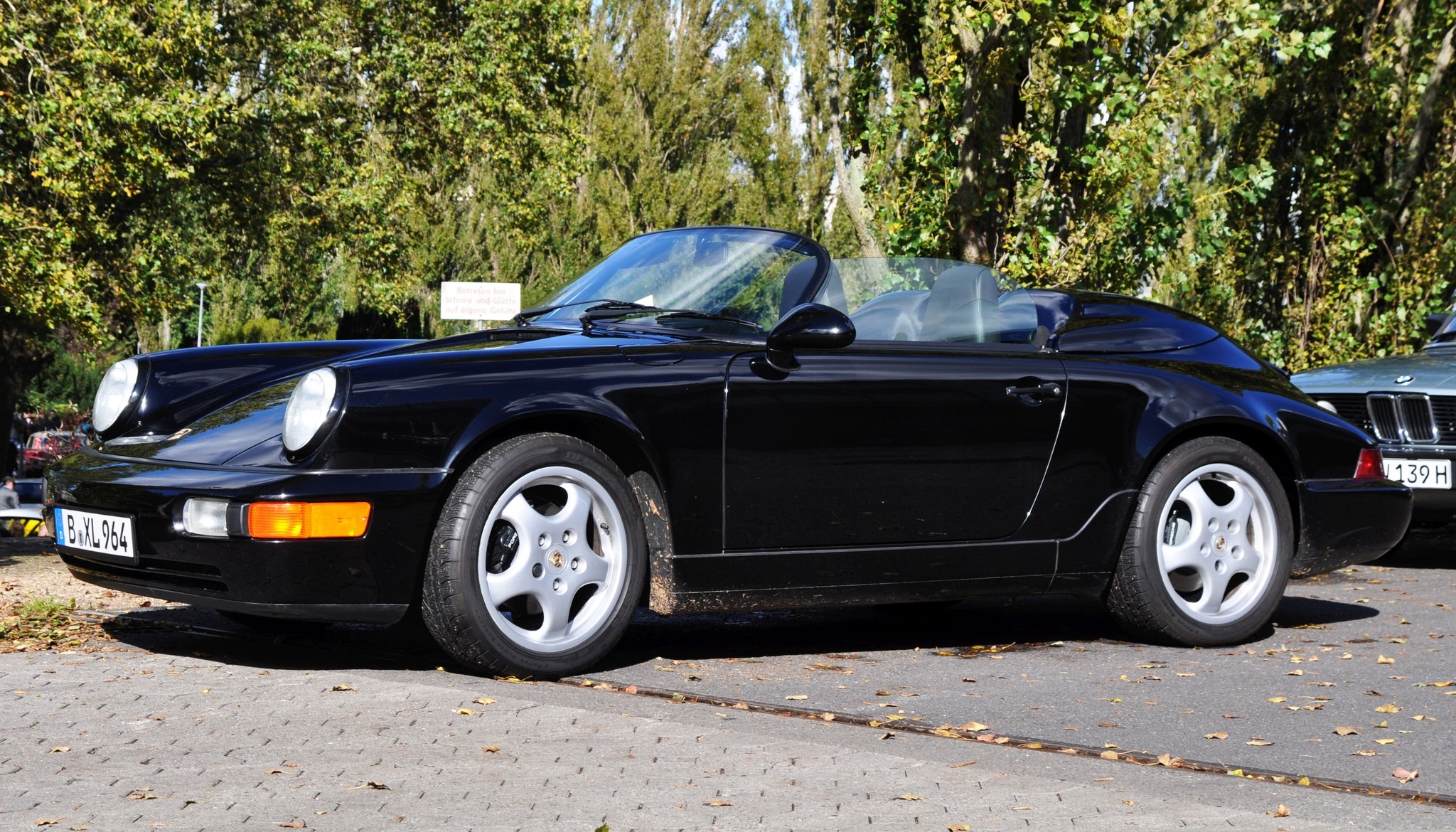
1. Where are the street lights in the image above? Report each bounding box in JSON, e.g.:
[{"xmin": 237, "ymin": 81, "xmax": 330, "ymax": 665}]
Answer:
[{"xmin": 194, "ymin": 282, "xmax": 208, "ymax": 347}]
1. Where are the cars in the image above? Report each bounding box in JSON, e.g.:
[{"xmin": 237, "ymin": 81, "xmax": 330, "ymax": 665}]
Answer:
[
  {"xmin": 0, "ymin": 428, "xmax": 91, "ymax": 539},
  {"xmin": 1286, "ymin": 308, "xmax": 1456, "ymax": 566}
]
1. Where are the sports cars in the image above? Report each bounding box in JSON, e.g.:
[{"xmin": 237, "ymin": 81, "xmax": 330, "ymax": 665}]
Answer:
[{"xmin": 52, "ymin": 220, "xmax": 1414, "ymax": 681}]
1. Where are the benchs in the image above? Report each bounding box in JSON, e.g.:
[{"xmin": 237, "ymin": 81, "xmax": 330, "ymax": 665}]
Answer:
[{"xmin": 848, "ymin": 290, "xmax": 1074, "ymax": 346}]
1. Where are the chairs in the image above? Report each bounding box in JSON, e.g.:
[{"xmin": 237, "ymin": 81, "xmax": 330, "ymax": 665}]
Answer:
[
  {"xmin": 918, "ymin": 264, "xmax": 1001, "ymax": 342},
  {"xmin": 778, "ymin": 256, "xmax": 849, "ymax": 321}
]
